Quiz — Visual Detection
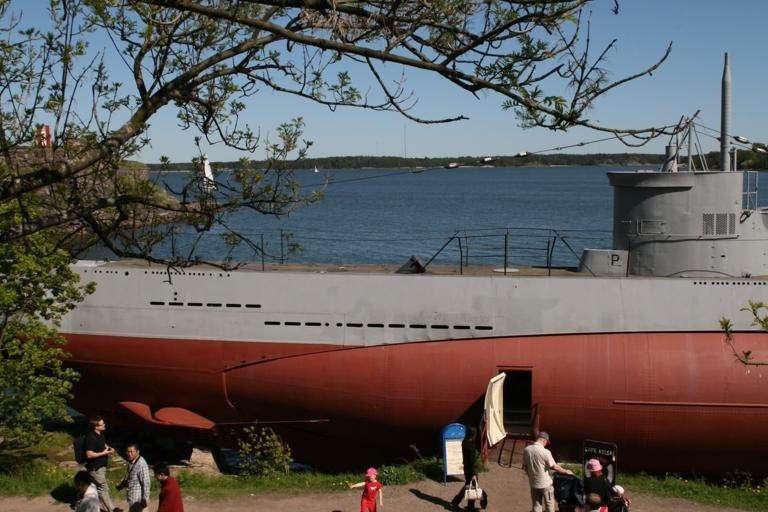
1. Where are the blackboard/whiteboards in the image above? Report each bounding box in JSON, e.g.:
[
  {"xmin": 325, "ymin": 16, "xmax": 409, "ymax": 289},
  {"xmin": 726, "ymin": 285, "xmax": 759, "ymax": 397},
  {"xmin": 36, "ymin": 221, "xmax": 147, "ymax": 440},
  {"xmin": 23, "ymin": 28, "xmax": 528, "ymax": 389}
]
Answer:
[{"xmin": 443, "ymin": 438, "xmax": 465, "ymax": 476}]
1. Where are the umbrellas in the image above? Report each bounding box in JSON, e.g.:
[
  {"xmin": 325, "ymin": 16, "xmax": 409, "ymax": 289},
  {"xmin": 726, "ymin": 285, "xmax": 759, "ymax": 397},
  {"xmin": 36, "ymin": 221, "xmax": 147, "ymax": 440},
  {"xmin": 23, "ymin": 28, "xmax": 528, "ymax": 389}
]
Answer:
[{"xmin": 198, "ymin": 152, "xmax": 217, "ymax": 196}]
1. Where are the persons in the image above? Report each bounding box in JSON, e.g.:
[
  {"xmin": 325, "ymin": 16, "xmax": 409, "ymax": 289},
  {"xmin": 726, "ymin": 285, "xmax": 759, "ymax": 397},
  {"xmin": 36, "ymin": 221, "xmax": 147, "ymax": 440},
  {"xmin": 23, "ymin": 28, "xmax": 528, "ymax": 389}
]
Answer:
[
  {"xmin": 448, "ymin": 424, "xmax": 478, "ymax": 511},
  {"xmin": 72, "ymin": 470, "xmax": 99, "ymax": 511},
  {"xmin": 574, "ymin": 492, "xmax": 609, "ymax": 511},
  {"xmin": 115, "ymin": 443, "xmax": 151, "ymax": 512},
  {"xmin": 151, "ymin": 463, "xmax": 183, "ymax": 512},
  {"xmin": 585, "ymin": 459, "xmax": 631, "ymax": 509},
  {"xmin": 82, "ymin": 413, "xmax": 124, "ymax": 511},
  {"xmin": 520, "ymin": 430, "xmax": 573, "ymax": 511},
  {"xmin": 346, "ymin": 466, "xmax": 383, "ymax": 512}
]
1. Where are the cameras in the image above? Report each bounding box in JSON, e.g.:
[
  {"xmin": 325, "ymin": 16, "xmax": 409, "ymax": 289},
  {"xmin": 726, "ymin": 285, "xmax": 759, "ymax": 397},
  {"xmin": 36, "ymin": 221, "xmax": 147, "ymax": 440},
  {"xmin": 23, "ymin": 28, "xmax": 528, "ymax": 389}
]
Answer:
[{"xmin": 116, "ymin": 480, "xmax": 129, "ymax": 491}]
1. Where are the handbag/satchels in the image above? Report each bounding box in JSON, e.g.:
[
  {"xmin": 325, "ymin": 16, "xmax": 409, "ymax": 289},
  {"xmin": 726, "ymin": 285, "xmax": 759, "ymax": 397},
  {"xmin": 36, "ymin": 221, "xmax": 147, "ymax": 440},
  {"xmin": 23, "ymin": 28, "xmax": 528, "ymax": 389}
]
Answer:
[{"xmin": 465, "ymin": 488, "xmax": 483, "ymax": 500}]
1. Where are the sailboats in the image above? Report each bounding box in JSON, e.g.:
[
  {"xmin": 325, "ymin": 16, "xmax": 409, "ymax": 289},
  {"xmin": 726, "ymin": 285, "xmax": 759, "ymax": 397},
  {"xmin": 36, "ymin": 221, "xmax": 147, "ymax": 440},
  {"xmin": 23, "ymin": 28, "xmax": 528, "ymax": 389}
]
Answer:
[
  {"xmin": 314, "ymin": 164, "xmax": 319, "ymax": 173},
  {"xmin": 200, "ymin": 152, "xmax": 217, "ymax": 190}
]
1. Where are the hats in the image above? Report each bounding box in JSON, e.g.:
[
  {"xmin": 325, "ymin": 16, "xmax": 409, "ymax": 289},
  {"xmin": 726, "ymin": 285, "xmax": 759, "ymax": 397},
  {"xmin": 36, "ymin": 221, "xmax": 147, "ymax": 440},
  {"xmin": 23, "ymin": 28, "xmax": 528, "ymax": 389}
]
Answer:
[
  {"xmin": 365, "ymin": 467, "xmax": 378, "ymax": 477},
  {"xmin": 612, "ymin": 484, "xmax": 625, "ymax": 497},
  {"xmin": 585, "ymin": 493, "xmax": 601, "ymax": 508},
  {"xmin": 538, "ymin": 432, "xmax": 551, "ymax": 445},
  {"xmin": 586, "ymin": 458, "xmax": 603, "ymax": 472}
]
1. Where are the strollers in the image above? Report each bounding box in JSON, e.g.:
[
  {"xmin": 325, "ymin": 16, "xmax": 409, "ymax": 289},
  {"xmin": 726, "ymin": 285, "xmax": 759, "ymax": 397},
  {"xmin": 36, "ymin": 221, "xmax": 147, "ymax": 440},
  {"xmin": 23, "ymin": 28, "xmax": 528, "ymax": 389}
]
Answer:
[{"xmin": 552, "ymin": 471, "xmax": 584, "ymax": 512}]
